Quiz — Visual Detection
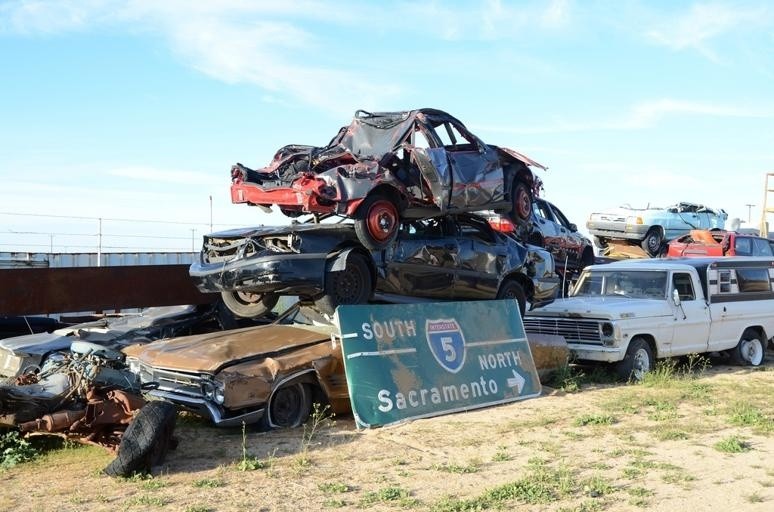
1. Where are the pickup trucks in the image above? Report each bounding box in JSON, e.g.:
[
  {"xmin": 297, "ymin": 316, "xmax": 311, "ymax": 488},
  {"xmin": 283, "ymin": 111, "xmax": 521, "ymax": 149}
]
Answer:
[{"xmin": 522, "ymin": 256, "xmax": 774, "ymax": 382}]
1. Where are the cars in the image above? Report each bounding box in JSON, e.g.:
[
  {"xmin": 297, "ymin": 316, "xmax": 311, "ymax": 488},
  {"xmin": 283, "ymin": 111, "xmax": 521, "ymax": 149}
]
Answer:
[
  {"xmin": 662, "ymin": 229, "xmax": 774, "ymax": 257},
  {"xmin": 0, "ymin": 305, "xmax": 223, "ymax": 423},
  {"xmin": 120, "ymin": 292, "xmax": 570, "ymax": 433},
  {"xmin": 471, "ymin": 197, "xmax": 594, "ymax": 269},
  {"xmin": 585, "ymin": 200, "xmax": 729, "ymax": 258},
  {"xmin": 189, "ymin": 211, "xmax": 561, "ymax": 320},
  {"xmin": 230, "ymin": 107, "xmax": 549, "ymax": 251}
]
